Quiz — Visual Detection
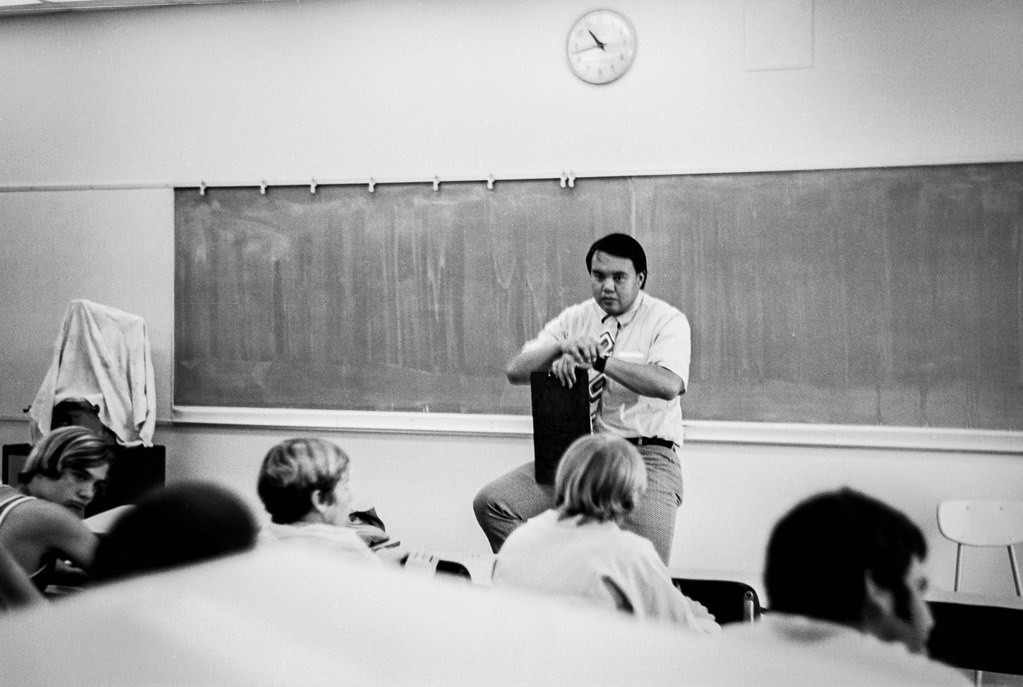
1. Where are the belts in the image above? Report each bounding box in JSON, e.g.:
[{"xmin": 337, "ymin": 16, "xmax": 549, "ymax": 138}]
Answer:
[{"xmin": 625, "ymin": 437, "xmax": 677, "ymax": 451}]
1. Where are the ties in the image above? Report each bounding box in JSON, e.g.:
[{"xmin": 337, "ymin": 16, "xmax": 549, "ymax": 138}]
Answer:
[{"xmin": 590, "ymin": 317, "xmax": 619, "ymax": 434}]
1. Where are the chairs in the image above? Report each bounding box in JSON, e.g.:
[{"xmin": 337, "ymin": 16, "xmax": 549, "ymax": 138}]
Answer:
[
  {"xmin": 3, "ymin": 302, "xmax": 145, "ymax": 486},
  {"xmin": 937, "ymin": 498, "xmax": 1023, "ymax": 687}
]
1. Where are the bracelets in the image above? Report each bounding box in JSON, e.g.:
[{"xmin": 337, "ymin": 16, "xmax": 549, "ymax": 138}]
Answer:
[{"xmin": 594, "ymin": 355, "xmax": 607, "ymax": 372}]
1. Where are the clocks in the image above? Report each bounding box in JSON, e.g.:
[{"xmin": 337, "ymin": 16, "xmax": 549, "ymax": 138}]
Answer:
[{"xmin": 564, "ymin": 8, "xmax": 636, "ymax": 85}]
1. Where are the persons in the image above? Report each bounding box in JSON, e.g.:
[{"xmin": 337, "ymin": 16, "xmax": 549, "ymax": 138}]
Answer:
[
  {"xmin": 724, "ymin": 488, "xmax": 973, "ymax": 687},
  {"xmin": 252, "ymin": 437, "xmax": 439, "ymax": 579},
  {"xmin": 474, "ymin": 232, "xmax": 691, "ymax": 566},
  {"xmin": 483, "ymin": 432, "xmax": 720, "ymax": 636},
  {"xmin": 0, "ymin": 426, "xmax": 257, "ymax": 613}
]
